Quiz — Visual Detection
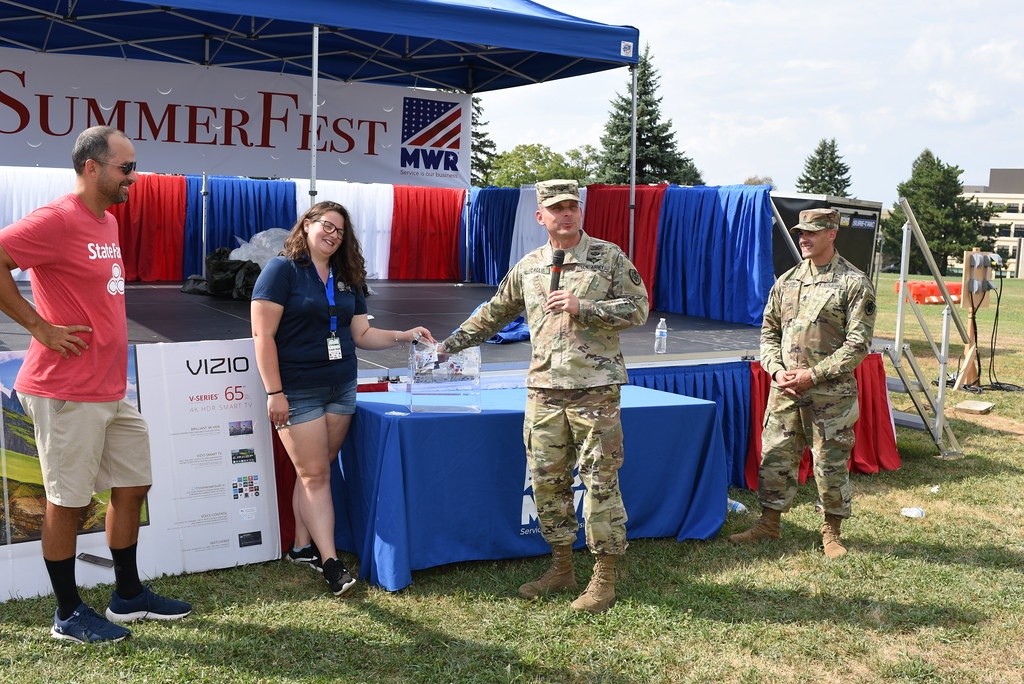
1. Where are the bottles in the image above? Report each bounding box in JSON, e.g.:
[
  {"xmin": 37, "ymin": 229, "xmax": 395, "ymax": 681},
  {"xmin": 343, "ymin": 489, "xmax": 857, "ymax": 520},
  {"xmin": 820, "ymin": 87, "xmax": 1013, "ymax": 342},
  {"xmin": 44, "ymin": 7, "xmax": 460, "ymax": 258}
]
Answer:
[
  {"xmin": 727, "ymin": 497, "xmax": 749, "ymax": 516},
  {"xmin": 655, "ymin": 318, "xmax": 668, "ymax": 353},
  {"xmin": 902, "ymin": 508, "xmax": 926, "ymax": 519}
]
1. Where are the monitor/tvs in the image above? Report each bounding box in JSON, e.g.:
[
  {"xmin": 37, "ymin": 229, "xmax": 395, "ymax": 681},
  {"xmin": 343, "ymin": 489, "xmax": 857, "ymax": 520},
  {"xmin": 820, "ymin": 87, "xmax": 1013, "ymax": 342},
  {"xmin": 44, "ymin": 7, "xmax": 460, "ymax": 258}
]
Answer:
[{"xmin": 0, "ymin": 344, "xmax": 150, "ymax": 546}]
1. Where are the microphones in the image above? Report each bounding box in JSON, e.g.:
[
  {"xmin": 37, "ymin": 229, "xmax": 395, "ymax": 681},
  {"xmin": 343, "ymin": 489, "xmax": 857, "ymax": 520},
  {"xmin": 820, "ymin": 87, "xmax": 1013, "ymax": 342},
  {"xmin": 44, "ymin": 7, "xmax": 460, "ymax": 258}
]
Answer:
[{"xmin": 549, "ymin": 249, "xmax": 565, "ymax": 293}]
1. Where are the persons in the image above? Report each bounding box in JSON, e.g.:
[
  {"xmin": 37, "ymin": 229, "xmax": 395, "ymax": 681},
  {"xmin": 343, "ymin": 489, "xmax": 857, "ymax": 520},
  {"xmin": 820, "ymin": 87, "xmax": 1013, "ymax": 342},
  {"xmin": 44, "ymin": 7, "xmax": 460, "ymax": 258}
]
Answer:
[
  {"xmin": 414, "ymin": 179, "xmax": 649, "ymax": 614},
  {"xmin": 729, "ymin": 208, "xmax": 877, "ymax": 560},
  {"xmin": 0, "ymin": 125, "xmax": 192, "ymax": 647},
  {"xmin": 251, "ymin": 201, "xmax": 438, "ymax": 597}
]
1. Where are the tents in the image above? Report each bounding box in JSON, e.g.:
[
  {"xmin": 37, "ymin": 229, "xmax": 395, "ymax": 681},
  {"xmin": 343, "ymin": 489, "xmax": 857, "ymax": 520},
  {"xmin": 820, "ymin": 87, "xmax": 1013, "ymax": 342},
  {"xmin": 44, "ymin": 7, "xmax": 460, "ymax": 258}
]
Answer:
[{"xmin": 0, "ymin": 0, "xmax": 639, "ymax": 284}]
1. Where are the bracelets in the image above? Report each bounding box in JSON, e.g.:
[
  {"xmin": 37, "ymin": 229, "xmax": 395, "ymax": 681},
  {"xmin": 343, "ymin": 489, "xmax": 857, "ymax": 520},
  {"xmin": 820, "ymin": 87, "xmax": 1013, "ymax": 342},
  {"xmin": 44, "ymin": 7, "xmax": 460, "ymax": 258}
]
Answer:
[
  {"xmin": 395, "ymin": 331, "xmax": 407, "ymax": 349},
  {"xmin": 268, "ymin": 390, "xmax": 283, "ymax": 395}
]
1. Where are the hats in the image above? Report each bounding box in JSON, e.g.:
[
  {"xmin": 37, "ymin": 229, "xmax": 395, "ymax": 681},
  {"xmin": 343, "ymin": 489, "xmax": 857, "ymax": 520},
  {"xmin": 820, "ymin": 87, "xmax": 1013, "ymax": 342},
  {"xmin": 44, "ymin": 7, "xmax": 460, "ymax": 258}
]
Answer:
[
  {"xmin": 535, "ymin": 179, "xmax": 584, "ymax": 207},
  {"xmin": 790, "ymin": 208, "xmax": 841, "ymax": 233}
]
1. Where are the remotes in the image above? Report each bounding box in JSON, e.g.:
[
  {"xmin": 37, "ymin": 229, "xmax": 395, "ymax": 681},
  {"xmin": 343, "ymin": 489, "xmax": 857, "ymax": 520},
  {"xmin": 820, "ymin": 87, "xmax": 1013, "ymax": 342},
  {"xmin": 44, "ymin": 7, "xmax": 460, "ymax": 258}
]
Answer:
[{"xmin": 77, "ymin": 554, "xmax": 114, "ymax": 568}]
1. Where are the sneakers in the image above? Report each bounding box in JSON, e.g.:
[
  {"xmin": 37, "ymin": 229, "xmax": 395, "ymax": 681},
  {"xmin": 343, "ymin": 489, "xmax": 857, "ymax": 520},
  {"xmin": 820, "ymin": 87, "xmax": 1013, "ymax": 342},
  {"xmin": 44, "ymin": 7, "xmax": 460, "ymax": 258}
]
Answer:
[
  {"xmin": 50, "ymin": 601, "xmax": 130, "ymax": 646},
  {"xmin": 287, "ymin": 546, "xmax": 324, "ymax": 573},
  {"xmin": 322, "ymin": 557, "xmax": 356, "ymax": 596},
  {"xmin": 105, "ymin": 585, "xmax": 192, "ymax": 622}
]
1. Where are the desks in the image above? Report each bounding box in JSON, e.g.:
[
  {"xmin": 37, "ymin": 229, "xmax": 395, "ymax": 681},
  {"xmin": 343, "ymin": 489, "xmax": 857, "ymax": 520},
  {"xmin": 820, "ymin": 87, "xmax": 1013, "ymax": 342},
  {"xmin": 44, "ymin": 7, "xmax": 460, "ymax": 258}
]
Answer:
[{"xmin": 331, "ymin": 384, "xmax": 728, "ymax": 592}]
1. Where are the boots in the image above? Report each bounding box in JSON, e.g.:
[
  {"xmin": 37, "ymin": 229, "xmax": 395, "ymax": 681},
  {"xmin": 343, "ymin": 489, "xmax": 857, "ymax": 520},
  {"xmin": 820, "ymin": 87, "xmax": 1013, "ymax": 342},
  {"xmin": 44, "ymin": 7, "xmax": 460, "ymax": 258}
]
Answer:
[
  {"xmin": 572, "ymin": 554, "xmax": 617, "ymax": 612},
  {"xmin": 820, "ymin": 514, "xmax": 847, "ymax": 558},
  {"xmin": 518, "ymin": 546, "xmax": 577, "ymax": 598},
  {"xmin": 729, "ymin": 508, "xmax": 784, "ymax": 543}
]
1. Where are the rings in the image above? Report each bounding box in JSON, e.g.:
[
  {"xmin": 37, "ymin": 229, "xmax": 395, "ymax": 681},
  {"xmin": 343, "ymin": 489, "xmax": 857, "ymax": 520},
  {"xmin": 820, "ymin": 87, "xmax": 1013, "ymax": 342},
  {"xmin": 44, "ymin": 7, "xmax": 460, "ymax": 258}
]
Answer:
[{"xmin": 558, "ymin": 302, "xmax": 560, "ymax": 305}]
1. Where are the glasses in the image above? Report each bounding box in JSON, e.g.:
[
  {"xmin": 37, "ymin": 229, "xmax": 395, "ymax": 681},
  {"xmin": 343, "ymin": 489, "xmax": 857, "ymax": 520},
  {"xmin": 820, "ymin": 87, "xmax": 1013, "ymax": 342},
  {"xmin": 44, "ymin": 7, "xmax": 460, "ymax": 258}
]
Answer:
[
  {"xmin": 96, "ymin": 160, "xmax": 136, "ymax": 175},
  {"xmin": 314, "ymin": 220, "xmax": 348, "ymax": 241}
]
